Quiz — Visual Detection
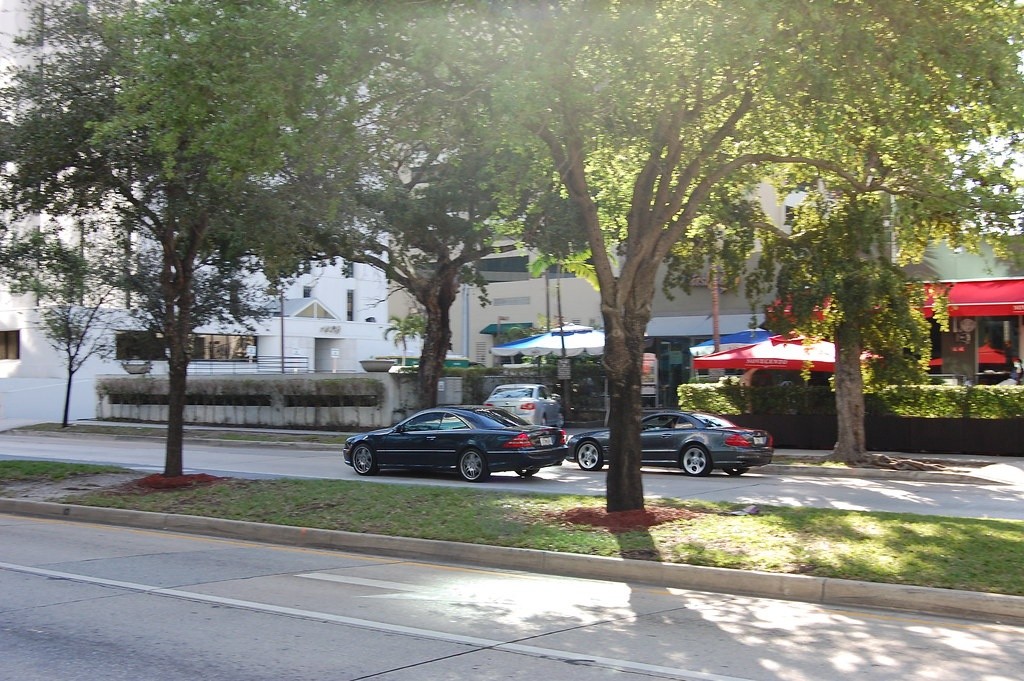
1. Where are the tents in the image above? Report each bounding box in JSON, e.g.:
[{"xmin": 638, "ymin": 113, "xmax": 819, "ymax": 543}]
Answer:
[
  {"xmin": 489, "ymin": 321, "xmax": 605, "ymax": 360},
  {"xmin": 689, "ymin": 327, "xmax": 884, "ymax": 373}
]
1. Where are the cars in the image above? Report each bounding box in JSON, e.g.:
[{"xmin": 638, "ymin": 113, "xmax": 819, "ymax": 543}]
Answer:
[
  {"xmin": 483, "ymin": 384, "xmax": 567, "ymax": 433},
  {"xmin": 342, "ymin": 404, "xmax": 569, "ymax": 482},
  {"xmin": 565, "ymin": 410, "xmax": 775, "ymax": 477}
]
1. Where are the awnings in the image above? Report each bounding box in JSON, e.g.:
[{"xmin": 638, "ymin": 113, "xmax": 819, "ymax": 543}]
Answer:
[
  {"xmin": 482, "ymin": 323, "xmax": 532, "ymax": 335},
  {"xmin": 644, "ymin": 314, "xmax": 766, "ymax": 337},
  {"xmin": 768, "ymin": 279, "xmax": 1024, "ymax": 321}
]
